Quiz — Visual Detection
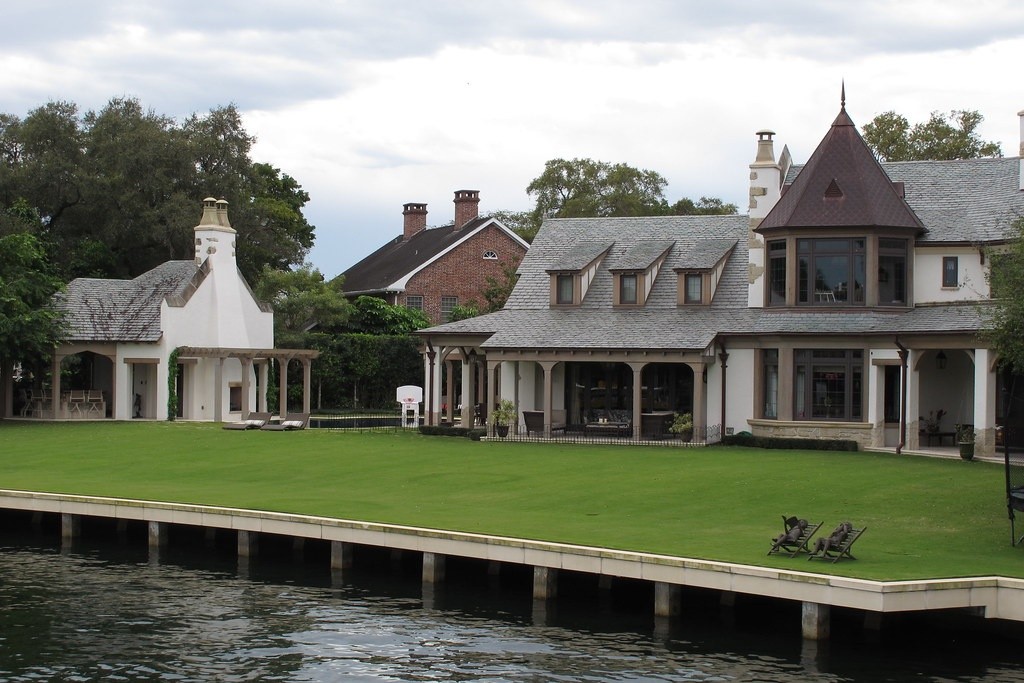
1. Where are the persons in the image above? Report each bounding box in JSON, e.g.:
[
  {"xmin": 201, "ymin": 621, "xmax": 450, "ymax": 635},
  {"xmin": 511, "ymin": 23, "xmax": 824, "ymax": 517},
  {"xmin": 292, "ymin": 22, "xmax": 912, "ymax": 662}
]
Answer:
[
  {"xmin": 808, "ymin": 522, "xmax": 852, "ymax": 558},
  {"xmin": 770, "ymin": 515, "xmax": 808, "ymax": 551}
]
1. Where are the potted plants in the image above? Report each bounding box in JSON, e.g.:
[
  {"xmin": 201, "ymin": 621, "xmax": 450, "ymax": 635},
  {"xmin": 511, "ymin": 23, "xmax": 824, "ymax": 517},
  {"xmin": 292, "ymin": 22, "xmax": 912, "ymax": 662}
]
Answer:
[
  {"xmin": 954, "ymin": 424, "xmax": 976, "ymax": 463},
  {"xmin": 919, "ymin": 410, "xmax": 947, "ymax": 432},
  {"xmin": 489, "ymin": 399, "xmax": 519, "ymax": 437},
  {"xmin": 669, "ymin": 412, "xmax": 693, "ymax": 442}
]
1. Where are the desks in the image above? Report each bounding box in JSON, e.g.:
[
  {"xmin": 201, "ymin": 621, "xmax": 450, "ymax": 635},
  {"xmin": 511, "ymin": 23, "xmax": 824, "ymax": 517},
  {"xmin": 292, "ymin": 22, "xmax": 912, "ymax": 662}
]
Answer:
[
  {"xmin": 642, "ymin": 411, "xmax": 674, "ymax": 441},
  {"xmin": 576, "ymin": 422, "xmax": 629, "ymax": 440},
  {"xmin": 919, "ymin": 432, "xmax": 956, "ymax": 447}
]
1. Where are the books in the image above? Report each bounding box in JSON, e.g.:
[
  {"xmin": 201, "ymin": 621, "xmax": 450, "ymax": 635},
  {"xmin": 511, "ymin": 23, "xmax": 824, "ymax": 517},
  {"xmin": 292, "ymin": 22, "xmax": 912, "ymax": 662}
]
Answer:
[{"xmin": 782, "ymin": 515, "xmax": 799, "ymax": 528}]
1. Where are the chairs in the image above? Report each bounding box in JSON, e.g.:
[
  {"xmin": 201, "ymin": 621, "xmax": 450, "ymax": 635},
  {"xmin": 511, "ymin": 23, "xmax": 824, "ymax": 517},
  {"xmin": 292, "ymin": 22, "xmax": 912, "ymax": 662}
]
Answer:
[
  {"xmin": 807, "ymin": 522, "xmax": 867, "ymax": 563},
  {"xmin": 260, "ymin": 411, "xmax": 311, "ymax": 431},
  {"xmin": 20, "ymin": 388, "xmax": 104, "ymax": 419},
  {"xmin": 222, "ymin": 412, "xmax": 273, "ymax": 431},
  {"xmin": 767, "ymin": 521, "xmax": 824, "ymax": 558}
]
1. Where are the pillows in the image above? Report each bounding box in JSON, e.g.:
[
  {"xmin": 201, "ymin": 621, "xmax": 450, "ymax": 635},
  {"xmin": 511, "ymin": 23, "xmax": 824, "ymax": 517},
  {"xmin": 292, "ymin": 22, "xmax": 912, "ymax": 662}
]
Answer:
[
  {"xmin": 281, "ymin": 420, "xmax": 303, "ymax": 427},
  {"xmin": 246, "ymin": 419, "xmax": 265, "ymax": 426}
]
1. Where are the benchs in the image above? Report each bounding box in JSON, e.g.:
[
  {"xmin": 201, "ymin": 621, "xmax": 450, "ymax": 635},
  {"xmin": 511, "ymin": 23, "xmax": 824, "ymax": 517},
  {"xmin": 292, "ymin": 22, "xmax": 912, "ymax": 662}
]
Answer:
[
  {"xmin": 583, "ymin": 408, "xmax": 632, "ymax": 439},
  {"xmin": 523, "ymin": 410, "xmax": 567, "ymax": 439}
]
